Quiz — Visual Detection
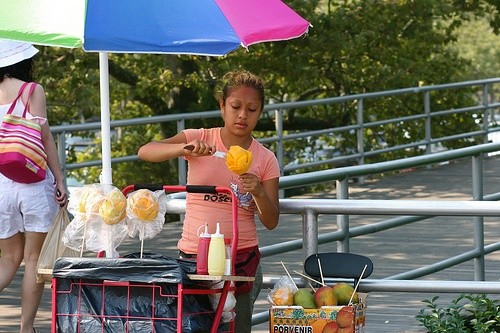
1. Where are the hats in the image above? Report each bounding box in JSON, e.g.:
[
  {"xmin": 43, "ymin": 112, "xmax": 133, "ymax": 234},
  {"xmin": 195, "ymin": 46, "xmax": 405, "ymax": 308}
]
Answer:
[{"xmin": 0, "ymin": 39, "xmax": 39, "ymax": 68}]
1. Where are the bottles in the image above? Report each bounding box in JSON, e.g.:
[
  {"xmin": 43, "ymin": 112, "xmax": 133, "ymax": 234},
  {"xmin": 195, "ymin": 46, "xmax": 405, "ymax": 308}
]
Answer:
[
  {"xmin": 196, "ymin": 224, "xmax": 211, "ymax": 274},
  {"xmin": 208, "ymin": 223, "xmax": 226, "ymax": 276}
]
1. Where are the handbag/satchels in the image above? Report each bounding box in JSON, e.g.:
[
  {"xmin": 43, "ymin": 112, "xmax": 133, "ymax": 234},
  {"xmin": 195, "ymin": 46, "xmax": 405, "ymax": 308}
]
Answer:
[
  {"xmin": 34, "ymin": 206, "xmax": 74, "ymax": 283},
  {"xmin": 0, "ymin": 82, "xmax": 48, "ymax": 184}
]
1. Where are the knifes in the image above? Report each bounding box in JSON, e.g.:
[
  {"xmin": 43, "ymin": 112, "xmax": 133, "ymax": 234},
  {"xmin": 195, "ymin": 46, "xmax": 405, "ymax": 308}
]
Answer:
[{"xmin": 183, "ymin": 144, "xmax": 227, "ymax": 158}]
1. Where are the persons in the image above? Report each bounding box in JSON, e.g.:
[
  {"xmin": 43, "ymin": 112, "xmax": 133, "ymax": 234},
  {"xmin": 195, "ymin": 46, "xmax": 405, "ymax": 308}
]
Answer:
[
  {"xmin": 138, "ymin": 68, "xmax": 279, "ymax": 333},
  {"xmin": 0, "ymin": 40, "xmax": 69, "ymax": 333}
]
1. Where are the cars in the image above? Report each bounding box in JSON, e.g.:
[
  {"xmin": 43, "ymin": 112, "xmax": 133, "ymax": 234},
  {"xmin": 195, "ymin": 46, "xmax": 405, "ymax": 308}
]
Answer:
[{"xmin": 283, "ymin": 130, "xmax": 428, "ymax": 194}]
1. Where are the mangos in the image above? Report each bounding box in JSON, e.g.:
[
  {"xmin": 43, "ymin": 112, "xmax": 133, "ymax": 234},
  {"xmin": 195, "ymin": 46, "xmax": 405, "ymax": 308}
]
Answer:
[
  {"xmin": 225, "ymin": 145, "xmax": 253, "ymax": 176},
  {"xmin": 273, "ymin": 282, "xmax": 360, "ymax": 309},
  {"xmin": 79, "ymin": 185, "xmax": 159, "ymax": 225}
]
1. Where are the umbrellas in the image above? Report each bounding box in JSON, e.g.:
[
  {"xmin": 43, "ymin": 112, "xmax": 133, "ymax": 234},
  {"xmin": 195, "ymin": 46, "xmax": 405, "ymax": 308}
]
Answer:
[{"xmin": 0, "ymin": 0, "xmax": 313, "ymax": 198}]
